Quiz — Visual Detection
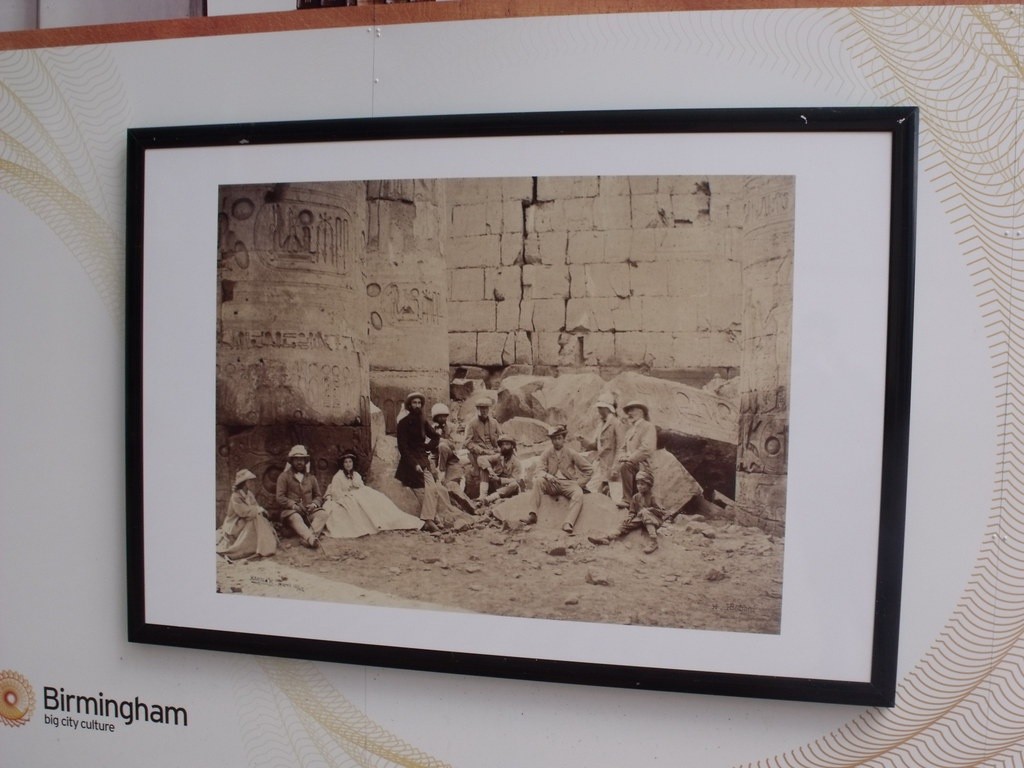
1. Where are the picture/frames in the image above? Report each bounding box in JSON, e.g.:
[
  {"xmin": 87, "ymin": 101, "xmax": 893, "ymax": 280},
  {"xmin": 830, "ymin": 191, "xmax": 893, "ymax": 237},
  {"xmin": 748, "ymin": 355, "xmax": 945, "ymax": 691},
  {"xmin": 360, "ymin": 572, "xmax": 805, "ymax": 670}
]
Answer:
[{"xmin": 125, "ymin": 105, "xmax": 922, "ymax": 707}]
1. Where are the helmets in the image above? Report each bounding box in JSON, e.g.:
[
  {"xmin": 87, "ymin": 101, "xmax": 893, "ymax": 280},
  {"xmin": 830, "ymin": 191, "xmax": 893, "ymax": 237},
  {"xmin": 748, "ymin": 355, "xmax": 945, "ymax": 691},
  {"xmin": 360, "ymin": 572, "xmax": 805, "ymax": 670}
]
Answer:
[{"xmin": 431, "ymin": 403, "xmax": 450, "ymax": 422}]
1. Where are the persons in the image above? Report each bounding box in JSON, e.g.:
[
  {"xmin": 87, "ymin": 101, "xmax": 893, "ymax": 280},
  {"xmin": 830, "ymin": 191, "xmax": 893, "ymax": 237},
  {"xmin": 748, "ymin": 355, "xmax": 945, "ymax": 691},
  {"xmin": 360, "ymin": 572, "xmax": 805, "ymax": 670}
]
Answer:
[
  {"xmin": 520, "ymin": 425, "xmax": 595, "ymax": 532},
  {"xmin": 274, "ymin": 444, "xmax": 330, "ymax": 548},
  {"xmin": 427, "ymin": 397, "xmax": 524, "ymax": 515},
  {"xmin": 392, "ymin": 391, "xmax": 442, "ymax": 530},
  {"xmin": 216, "ymin": 468, "xmax": 278, "ymax": 562},
  {"xmin": 578, "ymin": 400, "xmax": 667, "ymax": 554},
  {"xmin": 321, "ymin": 449, "xmax": 425, "ymax": 538}
]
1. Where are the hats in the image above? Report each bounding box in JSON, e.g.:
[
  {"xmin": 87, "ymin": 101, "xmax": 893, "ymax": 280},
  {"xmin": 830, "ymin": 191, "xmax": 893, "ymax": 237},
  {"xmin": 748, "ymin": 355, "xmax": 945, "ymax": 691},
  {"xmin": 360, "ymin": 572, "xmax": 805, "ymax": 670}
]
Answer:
[
  {"xmin": 475, "ymin": 397, "xmax": 492, "ymax": 407},
  {"xmin": 337, "ymin": 451, "xmax": 357, "ymax": 460},
  {"xmin": 234, "ymin": 469, "xmax": 257, "ymax": 486},
  {"xmin": 545, "ymin": 425, "xmax": 568, "ymax": 438},
  {"xmin": 497, "ymin": 434, "xmax": 516, "ymax": 449},
  {"xmin": 404, "ymin": 392, "xmax": 426, "ymax": 410},
  {"xmin": 593, "ymin": 393, "xmax": 616, "ymax": 413},
  {"xmin": 287, "ymin": 445, "xmax": 310, "ymax": 458},
  {"xmin": 622, "ymin": 400, "xmax": 649, "ymax": 417}
]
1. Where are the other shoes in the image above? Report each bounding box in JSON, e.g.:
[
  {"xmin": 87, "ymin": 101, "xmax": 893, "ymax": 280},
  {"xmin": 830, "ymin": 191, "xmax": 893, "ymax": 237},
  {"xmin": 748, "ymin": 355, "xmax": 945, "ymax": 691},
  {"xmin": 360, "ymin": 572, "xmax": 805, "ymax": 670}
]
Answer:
[
  {"xmin": 643, "ymin": 541, "xmax": 659, "ymax": 554},
  {"xmin": 483, "ymin": 496, "xmax": 490, "ymax": 505},
  {"xmin": 520, "ymin": 515, "xmax": 537, "ymax": 524},
  {"xmin": 616, "ymin": 500, "xmax": 630, "ymax": 508},
  {"xmin": 561, "ymin": 524, "xmax": 572, "ymax": 532},
  {"xmin": 588, "ymin": 535, "xmax": 609, "ymax": 545},
  {"xmin": 472, "ymin": 498, "xmax": 482, "ymax": 505},
  {"xmin": 298, "ymin": 537, "xmax": 319, "ymax": 548}
]
